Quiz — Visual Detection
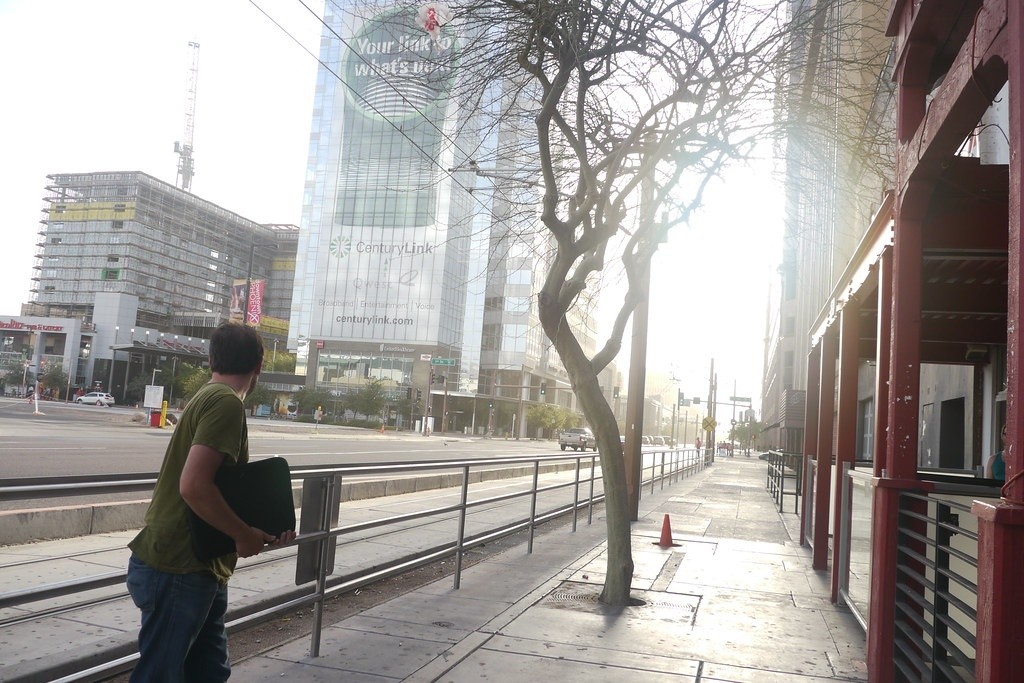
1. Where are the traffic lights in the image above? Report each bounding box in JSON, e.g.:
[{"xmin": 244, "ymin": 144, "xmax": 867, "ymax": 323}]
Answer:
[
  {"xmin": 405, "ymin": 388, "xmax": 411, "ymax": 398},
  {"xmin": 489, "ymin": 398, "xmax": 493, "ymax": 408},
  {"xmin": 541, "ymin": 382, "xmax": 545, "ymax": 394},
  {"xmin": 600, "ymin": 385, "xmax": 604, "ymax": 392},
  {"xmin": 431, "ymin": 373, "xmax": 435, "ymax": 383},
  {"xmin": 416, "ymin": 389, "xmax": 421, "ymax": 400},
  {"xmin": 438, "ymin": 375, "xmax": 442, "ymax": 382},
  {"xmin": 680, "ymin": 397, "xmax": 684, "ymax": 405},
  {"xmin": 613, "ymin": 386, "xmax": 619, "ymax": 398}
]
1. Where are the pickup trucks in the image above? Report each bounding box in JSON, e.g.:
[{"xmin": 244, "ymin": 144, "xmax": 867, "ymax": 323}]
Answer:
[{"xmin": 559, "ymin": 427, "xmax": 597, "ymax": 451}]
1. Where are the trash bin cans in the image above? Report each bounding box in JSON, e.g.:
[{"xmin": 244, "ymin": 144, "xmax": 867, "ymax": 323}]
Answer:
[{"xmin": 150, "ymin": 410, "xmax": 161, "ymax": 427}]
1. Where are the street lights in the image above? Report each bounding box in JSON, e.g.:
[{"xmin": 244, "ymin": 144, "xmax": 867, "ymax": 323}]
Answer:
[
  {"xmin": 242, "ymin": 241, "xmax": 279, "ymax": 323},
  {"xmin": 169, "ymin": 355, "xmax": 179, "ymax": 404},
  {"xmin": 271, "ymin": 338, "xmax": 278, "ymax": 373}
]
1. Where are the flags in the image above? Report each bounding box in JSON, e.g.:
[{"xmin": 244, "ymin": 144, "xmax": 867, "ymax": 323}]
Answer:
[
  {"xmin": 246, "ymin": 280, "xmax": 264, "ymax": 327},
  {"xmin": 228, "ymin": 279, "xmax": 246, "ymax": 324}
]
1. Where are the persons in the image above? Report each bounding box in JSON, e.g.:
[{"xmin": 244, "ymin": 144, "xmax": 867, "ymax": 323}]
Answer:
[
  {"xmin": 719, "ymin": 441, "xmax": 726, "ymax": 449},
  {"xmin": 127, "ymin": 323, "xmax": 297, "ymax": 683},
  {"xmin": 986, "ymin": 425, "xmax": 1006, "ymax": 481},
  {"xmin": 696, "ymin": 437, "xmax": 701, "ymax": 458}
]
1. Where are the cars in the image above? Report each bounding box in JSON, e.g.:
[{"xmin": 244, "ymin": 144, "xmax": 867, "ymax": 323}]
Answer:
[
  {"xmin": 619, "ymin": 435, "xmax": 677, "ymax": 446},
  {"xmin": 77, "ymin": 391, "xmax": 116, "ymax": 407}
]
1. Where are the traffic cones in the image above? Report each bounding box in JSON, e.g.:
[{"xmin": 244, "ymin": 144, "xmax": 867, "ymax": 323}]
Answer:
[
  {"xmin": 380, "ymin": 425, "xmax": 385, "ymax": 433},
  {"xmin": 659, "ymin": 514, "xmax": 672, "ymax": 546}
]
